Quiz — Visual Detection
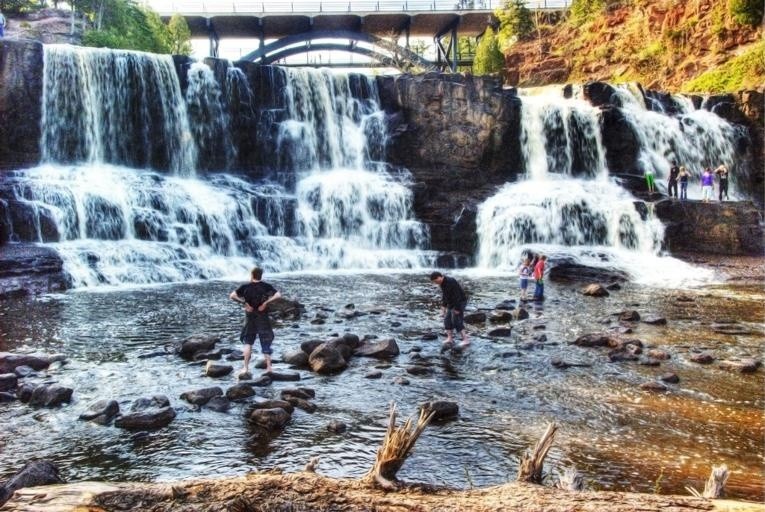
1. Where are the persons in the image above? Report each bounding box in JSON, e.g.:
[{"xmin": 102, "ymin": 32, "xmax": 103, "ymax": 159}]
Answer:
[
  {"xmin": 519, "ymin": 259, "xmax": 532, "ymax": 299},
  {"xmin": 714, "ymin": 164, "xmax": 730, "ymax": 201},
  {"xmin": 701, "ymin": 167, "xmax": 715, "ymax": 201},
  {"xmin": 533, "ymin": 255, "xmax": 547, "ymax": 299},
  {"xmin": 675, "ymin": 166, "xmax": 691, "ymax": 200},
  {"xmin": 709, "ymin": 169, "xmax": 716, "ymax": 201},
  {"xmin": 230, "ymin": 268, "xmax": 281, "ymax": 380},
  {"xmin": 0, "ymin": 9, "xmax": 7, "ymax": 37},
  {"xmin": 430, "ymin": 272, "xmax": 470, "ymax": 346},
  {"xmin": 667, "ymin": 161, "xmax": 679, "ymax": 199},
  {"xmin": 644, "ymin": 171, "xmax": 655, "ymax": 193}
]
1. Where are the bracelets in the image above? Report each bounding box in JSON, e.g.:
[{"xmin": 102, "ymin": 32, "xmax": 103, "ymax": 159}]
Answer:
[{"xmin": 243, "ymin": 301, "xmax": 246, "ymax": 306}]
